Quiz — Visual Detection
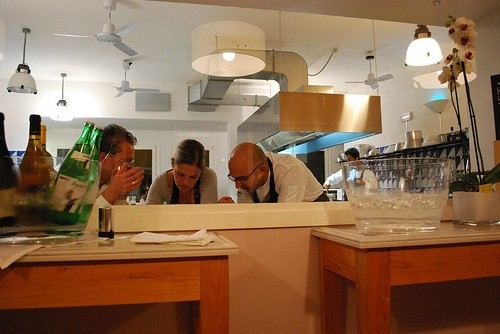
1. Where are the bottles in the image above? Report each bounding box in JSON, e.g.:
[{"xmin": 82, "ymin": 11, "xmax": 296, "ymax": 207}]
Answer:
[
  {"xmin": 18, "ymin": 115, "xmax": 106, "ymax": 236},
  {"xmin": 0, "ymin": 112, "xmax": 19, "ymax": 227},
  {"xmin": 99, "ymin": 207, "xmax": 115, "ymax": 239}
]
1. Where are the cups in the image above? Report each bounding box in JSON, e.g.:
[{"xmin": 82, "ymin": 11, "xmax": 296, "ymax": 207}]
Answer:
[
  {"xmin": 395, "ymin": 142, "xmax": 403, "ymax": 150},
  {"xmin": 441, "ymin": 135, "xmax": 447, "ymax": 143},
  {"xmin": 127, "ymin": 196, "xmax": 136, "ymax": 205}
]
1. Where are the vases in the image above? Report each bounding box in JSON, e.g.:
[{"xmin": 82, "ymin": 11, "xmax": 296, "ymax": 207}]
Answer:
[{"xmin": 452, "ymin": 191, "xmax": 500, "ymax": 226}]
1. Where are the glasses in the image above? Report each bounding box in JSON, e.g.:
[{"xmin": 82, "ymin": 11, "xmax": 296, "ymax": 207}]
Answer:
[
  {"xmin": 109, "ymin": 154, "xmax": 135, "ymax": 165},
  {"xmin": 227, "ymin": 163, "xmax": 264, "ymax": 182}
]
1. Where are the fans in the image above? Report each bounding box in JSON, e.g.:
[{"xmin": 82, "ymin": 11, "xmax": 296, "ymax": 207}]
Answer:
[
  {"xmin": 53, "ymin": 0, "xmax": 138, "ymax": 56},
  {"xmin": 98, "ymin": 61, "xmax": 159, "ymax": 98},
  {"xmin": 345, "ymin": 51, "xmax": 395, "ymax": 89}
]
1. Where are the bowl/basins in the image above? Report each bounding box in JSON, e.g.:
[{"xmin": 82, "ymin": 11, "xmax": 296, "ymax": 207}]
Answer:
[
  {"xmin": 342, "ymin": 158, "xmax": 450, "ymax": 234},
  {"xmin": 0, "ymin": 155, "xmax": 101, "ymax": 245},
  {"xmin": 368, "ymin": 148, "xmax": 381, "ymax": 156}
]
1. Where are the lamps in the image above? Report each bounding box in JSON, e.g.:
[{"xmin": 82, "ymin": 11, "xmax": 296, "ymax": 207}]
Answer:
[
  {"xmin": 56, "ymin": 73, "xmax": 67, "ymax": 106},
  {"xmin": 191, "ymin": 20, "xmax": 266, "ymax": 77},
  {"xmin": 7, "ymin": 28, "xmax": 38, "ymax": 94},
  {"xmin": 404, "ymin": 24, "xmax": 444, "ymax": 67},
  {"xmin": 424, "ymin": 99, "xmax": 448, "ymax": 114}
]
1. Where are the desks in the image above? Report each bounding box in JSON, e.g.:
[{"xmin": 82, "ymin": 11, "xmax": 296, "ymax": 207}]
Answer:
[
  {"xmin": 0, "ymin": 234, "xmax": 239, "ymax": 334},
  {"xmin": 310, "ymin": 226, "xmax": 500, "ymax": 334}
]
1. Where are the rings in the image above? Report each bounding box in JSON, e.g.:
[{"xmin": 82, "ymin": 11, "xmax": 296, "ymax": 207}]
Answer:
[{"xmin": 131, "ymin": 180, "xmax": 138, "ymax": 186}]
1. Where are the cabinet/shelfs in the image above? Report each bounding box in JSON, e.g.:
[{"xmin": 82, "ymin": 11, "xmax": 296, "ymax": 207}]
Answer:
[{"xmin": 336, "ymin": 139, "xmax": 471, "ymax": 195}]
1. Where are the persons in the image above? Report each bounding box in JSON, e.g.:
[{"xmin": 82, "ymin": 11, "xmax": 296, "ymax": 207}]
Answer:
[
  {"xmin": 219, "ymin": 141, "xmax": 332, "ymax": 203},
  {"xmin": 322, "ymin": 147, "xmax": 379, "ymax": 201},
  {"xmin": 55, "ymin": 121, "xmax": 145, "ymax": 231},
  {"xmin": 145, "ymin": 139, "xmax": 218, "ymax": 204}
]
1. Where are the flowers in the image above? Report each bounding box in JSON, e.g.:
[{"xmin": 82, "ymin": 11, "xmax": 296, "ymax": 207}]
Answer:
[{"xmin": 439, "ymin": 15, "xmax": 500, "ymax": 192}]
